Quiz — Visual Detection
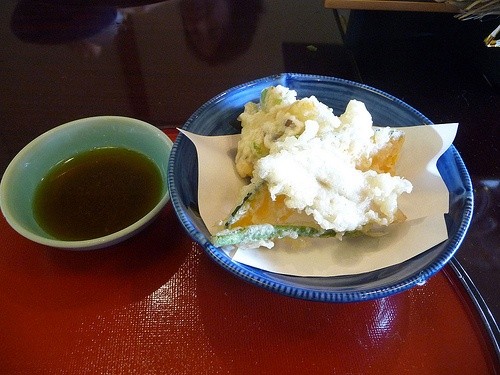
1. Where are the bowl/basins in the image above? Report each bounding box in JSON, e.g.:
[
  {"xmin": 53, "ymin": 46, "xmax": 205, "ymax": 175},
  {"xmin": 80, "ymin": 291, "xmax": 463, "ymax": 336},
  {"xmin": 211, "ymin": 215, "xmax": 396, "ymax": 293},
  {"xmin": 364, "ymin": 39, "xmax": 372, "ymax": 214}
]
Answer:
[
  {"xmin": 168, "ymin": 73, "xmax": 475, "ymax": 299},
  {"xmin": 1, "ymin": 114, "xmax": 174, "ymax": 251}
]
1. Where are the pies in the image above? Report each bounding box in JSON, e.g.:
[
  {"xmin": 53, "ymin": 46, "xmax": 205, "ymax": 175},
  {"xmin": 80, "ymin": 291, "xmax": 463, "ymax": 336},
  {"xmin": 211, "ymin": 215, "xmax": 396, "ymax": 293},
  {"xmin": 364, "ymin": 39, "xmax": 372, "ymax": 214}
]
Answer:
[{"xmin": 214, "ymin": 84, "xmax": 413, "ymax": 254}]
